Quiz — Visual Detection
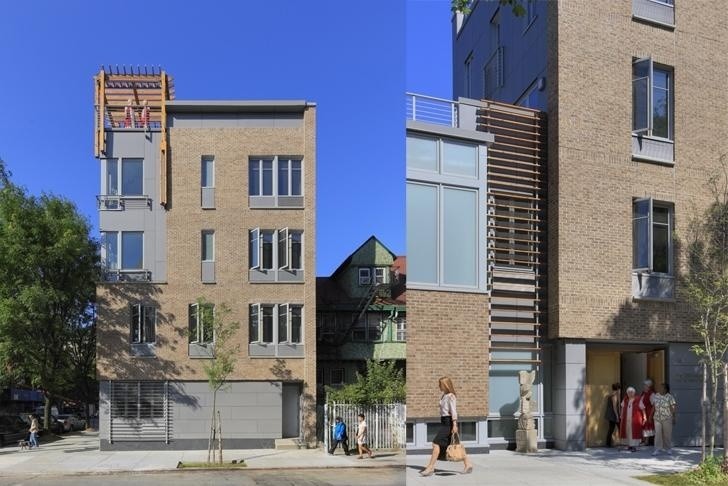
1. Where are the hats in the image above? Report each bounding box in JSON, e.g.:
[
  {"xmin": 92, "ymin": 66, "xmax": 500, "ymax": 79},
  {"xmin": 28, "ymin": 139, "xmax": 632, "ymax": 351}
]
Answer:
[{"xmin": 357, "ymin": 413, "xmax": 365, "ymax": 418}]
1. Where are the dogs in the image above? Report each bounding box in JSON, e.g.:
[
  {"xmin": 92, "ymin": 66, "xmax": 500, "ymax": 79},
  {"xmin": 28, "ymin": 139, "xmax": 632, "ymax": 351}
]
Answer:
[{"xmin": 18, "ymin": 441, "xmax": 33, "ymax": 450}]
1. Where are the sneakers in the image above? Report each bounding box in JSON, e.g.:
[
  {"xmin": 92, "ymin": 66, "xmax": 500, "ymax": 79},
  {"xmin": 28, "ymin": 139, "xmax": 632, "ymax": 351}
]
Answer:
[
  {"xmin": 652, "ymin": 447, "xmax": 663, "ymax": 457},
  {"xmin": 608, "ymin": 441, "xmax": 649, "ymax": 452},
  {"xmin": 356, "ymin": 454, "xmax": 364, "ymax": 460},
  {"xmin": 666, "ymin": 447, "xmax": 673, "ymax": 455},
  {"xmin": 368, "ymin": 449, "xmax": 372, "ymax": 457}
]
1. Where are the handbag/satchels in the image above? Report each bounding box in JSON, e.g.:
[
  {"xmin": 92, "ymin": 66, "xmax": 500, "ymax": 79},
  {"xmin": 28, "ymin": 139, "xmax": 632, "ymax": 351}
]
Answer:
[{"xmin": 444, "ymin": 431, "xmax": 470, "ymax": 463}]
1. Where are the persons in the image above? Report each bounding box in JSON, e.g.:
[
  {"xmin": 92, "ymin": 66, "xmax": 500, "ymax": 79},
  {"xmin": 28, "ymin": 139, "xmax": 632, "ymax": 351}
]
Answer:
[
  {"xmin": 604, "ymin": 382, "xmax": 621, "ymax": 447},
  {"xmin": 650, "ymin": 383, "xmax": 677, "ymax": 455},
  {"xmin": 619, "ymin": 385, "xmax": 647, "ymax": 452},
  {"xmin": 328, "ymin": 416, "xmax": 348, "ymax": 455},
  {"xmin": 123, "ymin": 95, "xmax": 135, "ymax": 128},
  {"xmin": 138, "ymin": 98, "xmax": 151, "ymax": 128},
  {"xmin": 640, "ymin": 378, "xmax": 655, "ymax": 446},
  {"xmin": 354, "ymin": 414, "xmax": 371, "ymax": 458},
  {"xmin": 418, "ymin": 375, "xmax": 471, "ymax": 476},
  {"xmin": 27, "ymin": 414, "xmax": 39, "ymax": 448}
]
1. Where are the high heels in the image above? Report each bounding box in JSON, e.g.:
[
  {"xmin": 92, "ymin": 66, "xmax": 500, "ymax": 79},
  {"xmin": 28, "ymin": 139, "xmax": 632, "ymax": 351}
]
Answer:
[
  {"xmin": 458, "ymin": 464, "xmax": 475, "ymax": 474},
  {"xmin": 420, "ymin": 468, "xmax": 435, "ymax": 477}
]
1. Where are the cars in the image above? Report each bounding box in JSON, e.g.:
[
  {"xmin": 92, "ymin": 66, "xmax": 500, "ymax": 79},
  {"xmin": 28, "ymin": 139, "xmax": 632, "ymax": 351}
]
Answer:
[{"xmin": 0, "ymin": 403, "xmax": 98, "ymax": 446}]
